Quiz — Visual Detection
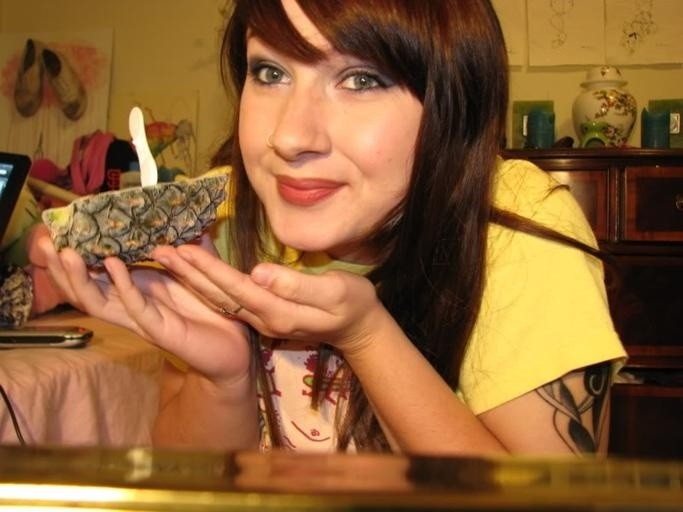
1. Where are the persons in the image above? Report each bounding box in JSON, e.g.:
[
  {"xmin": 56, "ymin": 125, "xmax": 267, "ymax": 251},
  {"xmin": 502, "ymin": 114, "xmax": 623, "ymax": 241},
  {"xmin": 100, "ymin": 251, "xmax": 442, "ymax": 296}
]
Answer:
[{"xmin": 33, "ymin": 1, "xmax": 629, "ymax": 456}]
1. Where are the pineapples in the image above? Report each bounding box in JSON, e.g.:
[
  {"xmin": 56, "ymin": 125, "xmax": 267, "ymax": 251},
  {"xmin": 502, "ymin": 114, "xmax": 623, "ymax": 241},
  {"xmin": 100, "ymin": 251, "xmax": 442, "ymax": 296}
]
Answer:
[{"xmin": 42, "ymin": 165, "xmax": 231, "ymax": 270}]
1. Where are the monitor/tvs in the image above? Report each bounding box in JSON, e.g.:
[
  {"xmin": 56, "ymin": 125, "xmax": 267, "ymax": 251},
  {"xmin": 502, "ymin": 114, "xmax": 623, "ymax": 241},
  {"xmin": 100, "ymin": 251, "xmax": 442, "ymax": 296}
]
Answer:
[{"xmin": 0, "ymin": 151, "xmax": 33, "ymax": 239}]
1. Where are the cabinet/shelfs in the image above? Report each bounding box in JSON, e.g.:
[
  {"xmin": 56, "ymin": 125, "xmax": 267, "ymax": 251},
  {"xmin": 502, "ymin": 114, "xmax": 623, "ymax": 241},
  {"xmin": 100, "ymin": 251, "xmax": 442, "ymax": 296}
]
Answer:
[{"xmin": 496, "ymin": 144, "xmax": 683, "ymax": 457}]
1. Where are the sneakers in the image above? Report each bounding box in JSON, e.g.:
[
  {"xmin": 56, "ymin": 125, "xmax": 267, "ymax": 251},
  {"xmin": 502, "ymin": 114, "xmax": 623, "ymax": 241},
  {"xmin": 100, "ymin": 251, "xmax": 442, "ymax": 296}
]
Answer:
[
  {"xmin": 12, "ymin": 38, "xmax": 43, "ymax": 118},
  {"xmin": 40, "ymin": 48, "xmax": 88, "ymax": 122}
]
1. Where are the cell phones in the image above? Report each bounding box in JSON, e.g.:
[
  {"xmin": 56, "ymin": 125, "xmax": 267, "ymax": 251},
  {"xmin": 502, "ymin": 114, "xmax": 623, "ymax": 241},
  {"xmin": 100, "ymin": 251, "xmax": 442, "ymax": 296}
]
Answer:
[{"xmin": 0, "ymin": 326, "xmax": 93, "ymax": 349}]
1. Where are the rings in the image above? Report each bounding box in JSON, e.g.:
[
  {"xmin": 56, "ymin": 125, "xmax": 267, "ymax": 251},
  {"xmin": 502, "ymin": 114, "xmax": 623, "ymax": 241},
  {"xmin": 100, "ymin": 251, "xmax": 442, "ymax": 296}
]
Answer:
[{"xmin": 219, "ymin": 302, "xmax": 242, "ymax": 317}]
